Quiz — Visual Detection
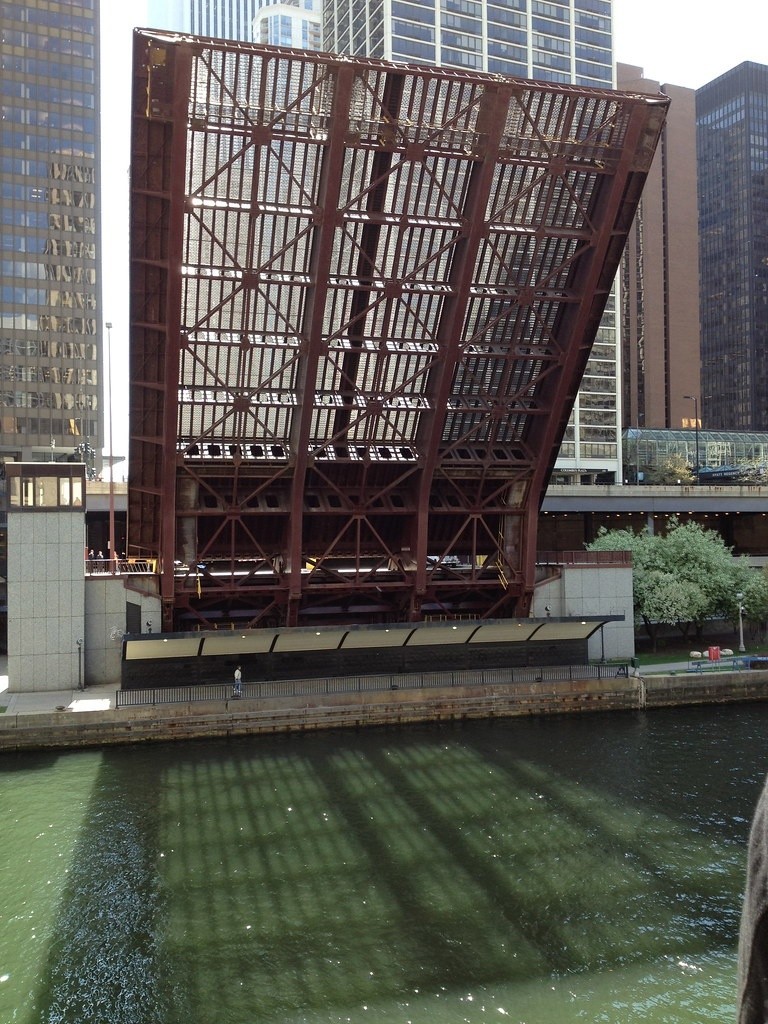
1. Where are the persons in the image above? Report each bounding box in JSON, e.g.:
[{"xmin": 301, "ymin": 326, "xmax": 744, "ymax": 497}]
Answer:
[
  {"xmin": 97, "ymin": 551, "xmax": 103, "ymax": 571},
  {"xmin": 234, "ymin": 665, "xmax": 242, "ymax": 693},
  {"xmin": 146, "ymin": 620, "xmax": 152, "ymax": 633},
  {"xmin": 114, "ymin": 551, "xmax": 119, "ymax": 570},
  {"xmin": 119, "ymin": 552, "xmax": 128, "ymax": 571},
  {"xmin": 88, "ymin": 550, "xmax": 94, "ymax": 573}
]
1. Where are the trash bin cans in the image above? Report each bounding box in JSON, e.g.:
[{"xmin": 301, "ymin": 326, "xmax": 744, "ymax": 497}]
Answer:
[{"xmin": 709, "ymin": 646, "xmax": 720, "ymax": 661}]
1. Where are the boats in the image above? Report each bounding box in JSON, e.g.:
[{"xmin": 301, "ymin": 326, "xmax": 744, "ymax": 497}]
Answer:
[{"xmin": 686, "ymin": 655, "xmax": 768, "ymax": 673}]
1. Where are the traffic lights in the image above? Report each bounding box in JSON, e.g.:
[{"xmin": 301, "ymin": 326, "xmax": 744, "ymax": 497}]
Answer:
[
  {"xmin": 74, "ymin": 442, "xmax": 96, "ymax": 459},
  {"xmin": 88, "ymin": 465, "xmax": 97, "ymax": 481}
]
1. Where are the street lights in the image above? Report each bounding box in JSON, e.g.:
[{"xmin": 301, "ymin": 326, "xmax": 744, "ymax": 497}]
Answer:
[
  {"xmin": 76, "ymin": 637, "xmax": 84, "ymax": 691},
  {"xmin": 635, "ymin": 412, "xmax": 646, "ymax": 485},
  {"xmin": 104, "ymin": 321, "xmax": 123, "ymax": 573},
  {"xmin": 684, "ymin": 395, "xmax": 702, "ymax": 486},
  {"xmin": 736, "ymin": 592, "xmax": 746, "ymax": 653},
  {"xmin": 51, "ymin": 439, "xmax": 56, "ymax": 461}
]
